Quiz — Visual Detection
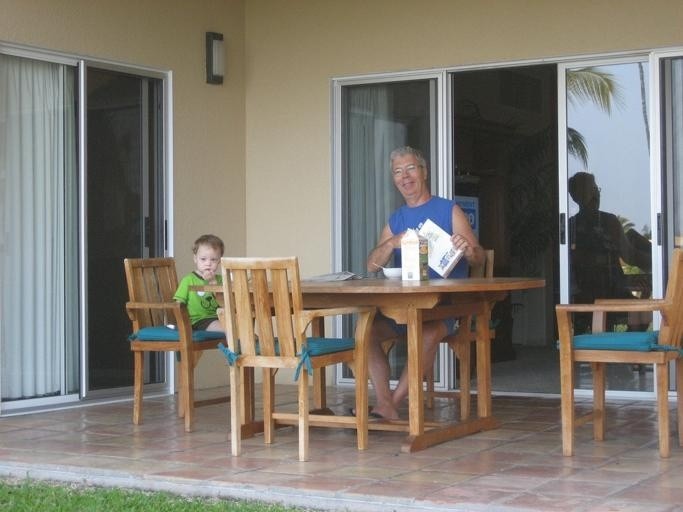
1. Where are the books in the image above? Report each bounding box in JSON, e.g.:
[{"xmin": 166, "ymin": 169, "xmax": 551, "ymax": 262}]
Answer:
[{"xmin": 417, "ymin": 218, "xmax": 465, "ymax": 279}]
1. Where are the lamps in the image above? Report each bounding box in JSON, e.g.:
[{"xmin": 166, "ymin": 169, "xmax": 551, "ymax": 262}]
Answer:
[{"xmin": 205, "ymin": 30, "xmax": 226, "ymax": 84}]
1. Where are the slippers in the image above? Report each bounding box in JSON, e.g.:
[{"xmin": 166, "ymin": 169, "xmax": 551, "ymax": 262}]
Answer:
[{"xmin": 349, "ymin": 405, "xmax": 388, "ymax": 432}]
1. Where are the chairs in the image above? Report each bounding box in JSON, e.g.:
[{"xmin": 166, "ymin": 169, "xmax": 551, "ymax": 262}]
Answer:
[
  {"xmin": 124, "ymin": 256, "xmax": 254, "ymax": 431},
  {"xmin": 553, "ymin": 249, "xmax": 683, "ymax": 458},
  {"xmin": 221, "ymin": 258, "xmax": 358, "ymax": 461},
  {"xmin": 374, "ymin": 247, "xmax": 499, "ymax": 428}
]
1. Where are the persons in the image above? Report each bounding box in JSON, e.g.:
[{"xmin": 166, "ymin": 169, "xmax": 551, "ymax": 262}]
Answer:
[
  {"xmin": 170, "ymin": 232, "xmax": 257, "ymax": 345},
  {"xmin": 567, "ymin": 171, "xmax": 653, "ymax": 332},
  {"xmin": 348, "ymin": 146, "xmax": 487, "ymax": 424}
]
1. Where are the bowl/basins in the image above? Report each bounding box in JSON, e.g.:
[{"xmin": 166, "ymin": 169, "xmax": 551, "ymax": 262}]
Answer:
[{"xmin": 382, "ymin": 267, "xmax": 403, "ymax": 279}]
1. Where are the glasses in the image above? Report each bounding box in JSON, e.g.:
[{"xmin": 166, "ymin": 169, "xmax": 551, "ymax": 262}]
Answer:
[{"xmin": 391, "ymin": 163, "xmax": 424, "ymax": 177}]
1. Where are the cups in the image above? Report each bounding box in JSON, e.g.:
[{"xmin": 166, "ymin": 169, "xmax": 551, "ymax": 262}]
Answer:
[{"xmin": 394, "ymin": 247, "xmax": 401, "ymax": 269}]
